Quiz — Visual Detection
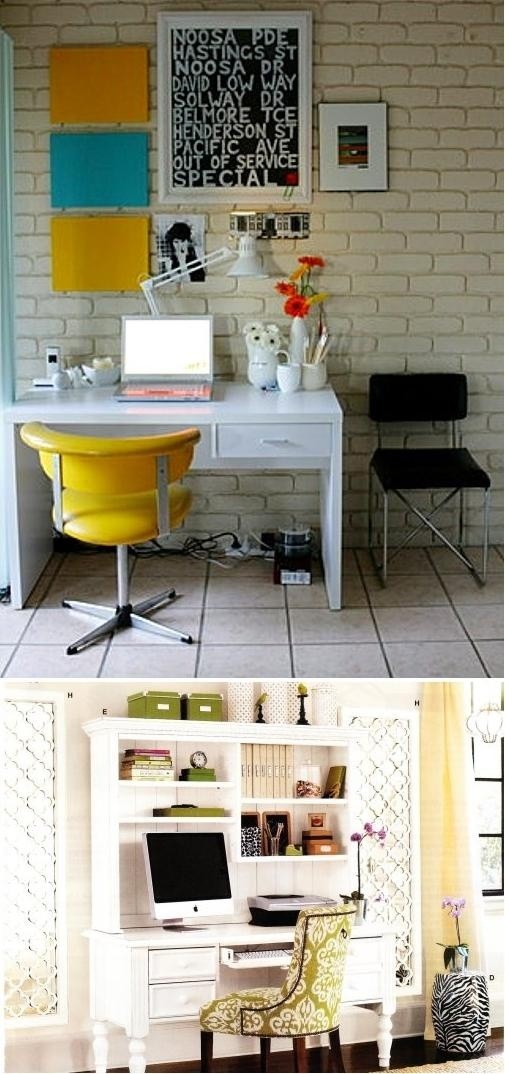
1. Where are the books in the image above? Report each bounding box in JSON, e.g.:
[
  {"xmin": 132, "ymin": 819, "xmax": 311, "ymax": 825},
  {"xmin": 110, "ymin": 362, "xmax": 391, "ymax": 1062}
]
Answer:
[
  {"xmin": 240, "ymin": 743, "xmax": 294, "ymax": 798},
  {"xmin": 321, "ymin": 766, "xmax": 345, "ymax": 799},
  {"xmin": 119, "ymin": 748, "xmax": 176, "ymax": 781}
]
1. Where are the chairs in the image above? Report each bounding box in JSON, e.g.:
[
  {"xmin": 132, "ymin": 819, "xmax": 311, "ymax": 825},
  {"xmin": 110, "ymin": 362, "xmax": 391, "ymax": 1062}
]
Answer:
[
  {"xmin": 370, "ymin": 373, "xmax": 492, "ymax": 588},
  {"xmin": 200, "ymin": 905, "xmax": 357, "ymax": 1072},
  {"xmin": 19, "ymin": 420, "xmax": 202, "ymax": 654}
]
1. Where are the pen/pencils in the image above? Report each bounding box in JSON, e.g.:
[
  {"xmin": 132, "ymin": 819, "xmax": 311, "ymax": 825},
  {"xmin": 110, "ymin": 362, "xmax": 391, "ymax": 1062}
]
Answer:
[
  {"xmin": 263, "ymin": 822, "xmax": 285, "ymax": 838},
  {"xmin": 302, "ymin": 323, "xmax": 337, "ymax": 364}
]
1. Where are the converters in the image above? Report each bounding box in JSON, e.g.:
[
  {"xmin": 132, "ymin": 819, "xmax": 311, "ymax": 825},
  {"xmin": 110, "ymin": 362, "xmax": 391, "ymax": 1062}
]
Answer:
[{"xmin": 260, "ymin": 532, "xmax": 276, "ymax": 551}]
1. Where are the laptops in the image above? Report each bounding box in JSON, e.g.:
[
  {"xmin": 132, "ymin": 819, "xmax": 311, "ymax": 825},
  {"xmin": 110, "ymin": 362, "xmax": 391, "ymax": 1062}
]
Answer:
[{"xmin": 112, "ymin": 313, "xmax": 215, "ymax": 402}]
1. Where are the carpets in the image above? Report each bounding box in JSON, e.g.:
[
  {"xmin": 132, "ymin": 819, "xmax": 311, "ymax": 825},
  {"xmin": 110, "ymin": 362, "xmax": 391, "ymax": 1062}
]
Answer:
[{"xmin": 370, "ymin": 1053, "xmax": 504, "ymax": 1071}]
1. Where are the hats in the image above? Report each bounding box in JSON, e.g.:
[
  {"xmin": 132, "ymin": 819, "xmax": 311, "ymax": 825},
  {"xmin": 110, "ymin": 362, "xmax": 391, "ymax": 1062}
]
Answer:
[{"xmin": 164, "ymin": 222, "xmax": 192, "ymax": 243}]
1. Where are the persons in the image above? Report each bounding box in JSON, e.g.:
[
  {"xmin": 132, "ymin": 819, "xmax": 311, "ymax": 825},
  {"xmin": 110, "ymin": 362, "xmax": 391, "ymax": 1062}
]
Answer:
[{"xmin": 162, "ymin": 223, "xmax": 205, "ymax": 281}]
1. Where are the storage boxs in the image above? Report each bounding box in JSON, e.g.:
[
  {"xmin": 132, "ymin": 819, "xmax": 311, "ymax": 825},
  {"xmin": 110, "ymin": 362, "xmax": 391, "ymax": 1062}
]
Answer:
[
  {"xmin": 182, "ymin": 692, "xmax": 224, "ymax": 721},
  {"xmin": 126, "ymin": 690, "xmax": 181, "ymax": 719}
]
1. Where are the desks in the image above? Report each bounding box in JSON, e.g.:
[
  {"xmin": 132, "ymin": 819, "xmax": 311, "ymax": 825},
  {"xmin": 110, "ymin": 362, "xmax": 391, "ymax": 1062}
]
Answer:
[
  {"xmin": 3, "ymin": 379, "xmax": 343, "ymax": 612},
  {"xmin": 81, "ymin": 928, "xmax": 404, "ymax": 1073},
  {"xmin": 431, "ymin": 972, "xmax": 490, "ymax": 1055}
]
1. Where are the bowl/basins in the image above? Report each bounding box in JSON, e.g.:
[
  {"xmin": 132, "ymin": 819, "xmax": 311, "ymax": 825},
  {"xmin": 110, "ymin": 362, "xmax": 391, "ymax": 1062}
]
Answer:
[{"xmin": 81, "ymin": 361, "xmax": 120, "ymax": 386}]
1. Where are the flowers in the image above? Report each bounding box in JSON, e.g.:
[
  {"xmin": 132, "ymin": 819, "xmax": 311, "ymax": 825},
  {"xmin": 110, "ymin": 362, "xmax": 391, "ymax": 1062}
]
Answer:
[
  {"xmin": 275, "ymin": 254, "xmax": 327, "ymax": 316},
  {"xmin": 340, "ymin": 822, "xmax": 388, "ymax": 897},
  {"xmin": 437, "ymin": 895, "xmax": 473, "ymax": 968},
  {"xmin": 242, "ymin": 319, "xmax": 282, "ymax": 352}
]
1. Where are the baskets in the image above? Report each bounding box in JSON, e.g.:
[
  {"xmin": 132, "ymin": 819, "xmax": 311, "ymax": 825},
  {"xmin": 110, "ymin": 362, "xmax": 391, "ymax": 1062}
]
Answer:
[{"xmin": 241, "ymin": 826, "xmax": 262, "ymax": 857}]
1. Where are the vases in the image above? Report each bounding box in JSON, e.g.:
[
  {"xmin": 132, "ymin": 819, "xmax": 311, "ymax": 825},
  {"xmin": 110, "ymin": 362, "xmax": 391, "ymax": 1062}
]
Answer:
[
  {"xmin": 289, "ymin": 316, "xmax": 310, "ymax": 365},
  {"xmin": 247, "ymin": 348, "xmax": 276, "ymax": 390},
  {"xmin": 343, "ymin": 894, "xmax": 368, "ymax": 926},
  {"xmin": 444, "ymin": 946, "xmax": 470, "ymax": 973}
]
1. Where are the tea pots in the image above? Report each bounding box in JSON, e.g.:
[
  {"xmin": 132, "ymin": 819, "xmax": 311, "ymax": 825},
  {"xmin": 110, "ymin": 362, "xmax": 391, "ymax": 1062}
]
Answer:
[{"xmin": 245, "ymin": 336, "xmax": 291, "ymax": 391}]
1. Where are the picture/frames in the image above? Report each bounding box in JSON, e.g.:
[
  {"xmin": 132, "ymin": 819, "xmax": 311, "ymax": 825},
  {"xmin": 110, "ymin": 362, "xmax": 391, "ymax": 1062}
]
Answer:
[
  {"xmin": 262, "ymin": 811, "xmax": 292, "ymax": 856},
  {"xmin": 241, "ymin": 812, "xmax": 260, "ymax": 831},
  {"xmin": 156, "ymin": 10, "xmax": 312, "ymax": 203},
  {"xmin": 318, "ymin": 102, "xmax": 390, "ymax": 190},
  {"xmin": 307, "ymin": 813, "xmax": 327, "ymax": 830}
]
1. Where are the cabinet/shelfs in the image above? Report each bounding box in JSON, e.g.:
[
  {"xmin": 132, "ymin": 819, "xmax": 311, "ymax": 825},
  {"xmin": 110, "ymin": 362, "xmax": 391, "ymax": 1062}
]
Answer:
[{"xmin": 79, "ymin": 716, "xmax": 354, "ymax": 933}]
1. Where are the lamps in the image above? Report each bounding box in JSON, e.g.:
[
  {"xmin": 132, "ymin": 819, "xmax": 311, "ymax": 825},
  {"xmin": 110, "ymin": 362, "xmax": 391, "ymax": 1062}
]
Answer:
[
  {"xmin": 139, "ymin": 235, "xmax": 271, "ymax": 316},
  {"xmin": 466, "ymin": 677, "xmax": 505, "ymax": 744}
]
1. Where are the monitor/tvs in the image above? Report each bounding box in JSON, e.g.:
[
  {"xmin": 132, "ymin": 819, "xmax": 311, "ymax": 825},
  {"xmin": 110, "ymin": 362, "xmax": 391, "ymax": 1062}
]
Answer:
[{"xmin": 141, "ymin": 832, "xmax": 236, "ymax": 933}]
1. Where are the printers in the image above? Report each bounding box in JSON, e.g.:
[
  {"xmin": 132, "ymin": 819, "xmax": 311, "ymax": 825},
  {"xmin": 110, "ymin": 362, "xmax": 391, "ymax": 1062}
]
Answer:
[{"xmin": 247, "ymin": 893, "xmax": 337, "ymax": 927}]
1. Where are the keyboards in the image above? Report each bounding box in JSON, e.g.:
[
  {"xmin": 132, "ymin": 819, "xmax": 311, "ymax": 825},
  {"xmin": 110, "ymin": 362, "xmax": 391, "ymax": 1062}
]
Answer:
[{"xmin": 236, "ymin": 947, "xmax": 295, "ymax": 962}]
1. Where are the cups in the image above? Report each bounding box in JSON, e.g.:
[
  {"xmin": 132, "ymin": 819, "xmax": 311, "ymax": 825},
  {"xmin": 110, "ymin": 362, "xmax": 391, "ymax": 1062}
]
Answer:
[
  {"xmin": 276, "ymin": 363, "xmax": 301, "ymax": 394},
  {"xmin": 302, "ymin": 360, "xmax": 327, "ymax": 391}
]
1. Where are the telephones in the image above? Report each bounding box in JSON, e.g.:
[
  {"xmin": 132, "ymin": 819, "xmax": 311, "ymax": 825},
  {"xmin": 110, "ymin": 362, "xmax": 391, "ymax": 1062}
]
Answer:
[{"xmin": 32, "ymin": 346, "xmax": 60, "ymax": 386}]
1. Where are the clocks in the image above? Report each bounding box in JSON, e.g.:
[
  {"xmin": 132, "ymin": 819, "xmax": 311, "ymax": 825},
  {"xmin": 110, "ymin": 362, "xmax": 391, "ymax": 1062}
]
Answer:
[{"xmin": 190, "ymin": 750, "xmax": 208, "ymax": 768}]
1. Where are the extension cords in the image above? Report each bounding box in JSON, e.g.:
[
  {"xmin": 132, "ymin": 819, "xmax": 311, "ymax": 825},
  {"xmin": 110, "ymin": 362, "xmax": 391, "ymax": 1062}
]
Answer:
[{"xmin": 224, "ymin": 546, "xmax": 275, "ymax": 557}]
